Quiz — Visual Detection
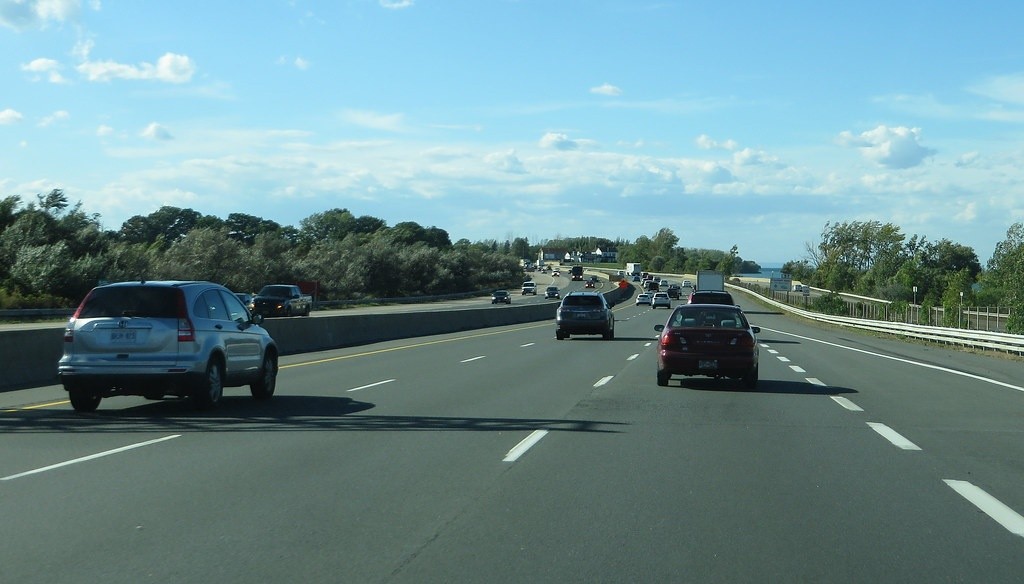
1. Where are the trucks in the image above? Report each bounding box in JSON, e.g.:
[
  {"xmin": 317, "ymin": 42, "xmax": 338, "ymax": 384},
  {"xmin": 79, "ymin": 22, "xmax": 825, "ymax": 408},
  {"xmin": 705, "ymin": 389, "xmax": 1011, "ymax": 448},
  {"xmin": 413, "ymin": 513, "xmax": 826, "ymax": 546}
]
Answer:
[{"xmin": 695, "ymin": 269, "xmax": 725, "ymax": 292}]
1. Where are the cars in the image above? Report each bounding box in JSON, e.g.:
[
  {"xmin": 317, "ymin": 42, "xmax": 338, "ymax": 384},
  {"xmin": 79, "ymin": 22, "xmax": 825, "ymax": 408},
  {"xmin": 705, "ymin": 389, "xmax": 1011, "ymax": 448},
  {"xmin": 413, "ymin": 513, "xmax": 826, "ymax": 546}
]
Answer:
[
  {"xmin": 231, "ymin": 293, "xmax": 255, "ymax": 314},
  {"xmin": 56, "ymin": 280, "xmax": 280, "ymax": 416},
  {"xmin": 524, "ymin": 255, "xmax": 682, "ymax": 301},
  {"xmin": 491, "ymin": 290, "xmax": 511, "ymax": 305},
  {"xmin": 555, "ymin": 290, "xmax": 614, "ymax": 341},
  {"xmin": 791, "ymin": 285, "xmax": 803, "ymax": 292},
  {"xmin": 654, "ymin": 304, "xmax": 762, "ymax": 391},
  {"xmin": 636, "ymin": 294, "xmax": 651, "ymax": 307},
  {"xmin": 252, "ymin": 285, "xmax": 313, "ymax": 319},
  {"xmin": 682, "ymin": 280, "xmax": 691, "ymax": 288},
  {"xmin": 653, "ymin": 292, "xmax": 671, "ymax": 309}
]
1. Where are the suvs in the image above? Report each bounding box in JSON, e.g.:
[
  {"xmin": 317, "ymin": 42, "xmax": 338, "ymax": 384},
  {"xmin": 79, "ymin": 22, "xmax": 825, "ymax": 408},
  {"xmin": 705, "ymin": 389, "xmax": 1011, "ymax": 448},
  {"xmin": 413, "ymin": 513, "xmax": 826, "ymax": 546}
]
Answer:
[
  {"xmin": 688, "ymin": 290, "xmax": 733, "ymax": 306},
  {"xmin": 521, "ymin": 282, "xmax": 538, "ymax": 296}
]
